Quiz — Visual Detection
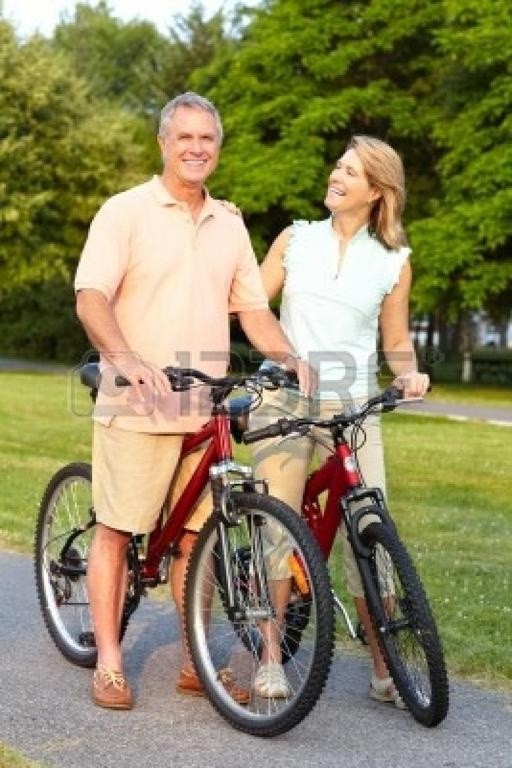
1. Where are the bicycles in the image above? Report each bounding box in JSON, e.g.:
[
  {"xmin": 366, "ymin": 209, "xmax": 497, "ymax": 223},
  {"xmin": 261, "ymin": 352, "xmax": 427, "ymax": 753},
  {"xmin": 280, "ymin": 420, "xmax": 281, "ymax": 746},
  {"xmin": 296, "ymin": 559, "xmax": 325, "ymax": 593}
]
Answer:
[
  {"xmin": 209, "ymin": 385, "xmax": 449, "ymax": 728},
  {"xmin": 31, "ymin": 367, "xmax": 336, "ymax": 740}
]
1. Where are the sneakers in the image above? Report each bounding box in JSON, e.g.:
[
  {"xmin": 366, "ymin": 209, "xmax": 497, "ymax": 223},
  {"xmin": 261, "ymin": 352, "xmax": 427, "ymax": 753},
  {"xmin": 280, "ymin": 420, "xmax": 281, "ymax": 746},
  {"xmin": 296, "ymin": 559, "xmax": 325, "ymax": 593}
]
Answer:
[
  {"xmin": 252, "ymin": 662, "xmax": 289, "ymax": 698},
  {"xmin": 369, "ymin": 672, "xmax": 430, "ymax": 713}
]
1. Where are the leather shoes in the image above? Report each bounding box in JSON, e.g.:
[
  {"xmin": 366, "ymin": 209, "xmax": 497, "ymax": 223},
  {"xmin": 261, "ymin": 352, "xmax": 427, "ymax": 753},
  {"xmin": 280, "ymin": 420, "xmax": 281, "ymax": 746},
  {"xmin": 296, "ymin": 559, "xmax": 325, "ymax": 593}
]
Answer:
[
  {"xmin": 176, "ymin": 669, "xmax": 250, "ymax": 704},
  {"xmin": 94, "ymin": 665, "xmax": 132, "ymax": 710}
]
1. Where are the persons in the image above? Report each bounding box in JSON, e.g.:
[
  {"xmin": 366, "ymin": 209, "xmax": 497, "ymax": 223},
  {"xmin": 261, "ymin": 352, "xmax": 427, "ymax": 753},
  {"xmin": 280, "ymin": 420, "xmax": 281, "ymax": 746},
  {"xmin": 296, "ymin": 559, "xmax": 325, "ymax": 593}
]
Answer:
[
  {"xmin": 73, "ymin": 92, "xmax": 320, "ymax": 711},
  {"xmin": 231, "ymin": 135, "xmax": 431, "ymax": 711}
]
